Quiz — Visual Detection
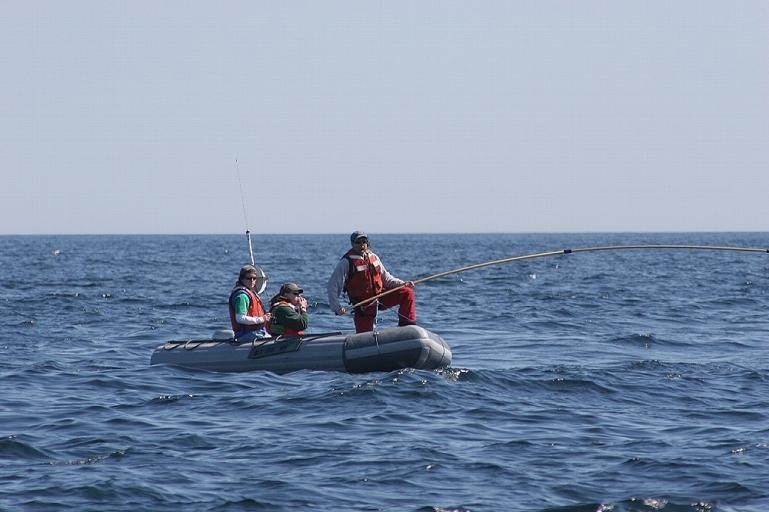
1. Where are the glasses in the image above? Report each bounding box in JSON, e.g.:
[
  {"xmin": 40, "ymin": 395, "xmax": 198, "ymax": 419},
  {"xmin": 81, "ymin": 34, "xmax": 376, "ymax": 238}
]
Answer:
[{"xmin": 246, "ymin": 276, "xmax": 257, "ymax": 280}]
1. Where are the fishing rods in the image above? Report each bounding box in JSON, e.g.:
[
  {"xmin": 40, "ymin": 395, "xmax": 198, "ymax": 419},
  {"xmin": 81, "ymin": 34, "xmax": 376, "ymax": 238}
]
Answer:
[
  {"xmin": 335, "ymin": 245, "xmax": 769, "ymax": 315},
  {"xmin": 236, "ymin": 159, "xmax": 255, "ymax": 266}
]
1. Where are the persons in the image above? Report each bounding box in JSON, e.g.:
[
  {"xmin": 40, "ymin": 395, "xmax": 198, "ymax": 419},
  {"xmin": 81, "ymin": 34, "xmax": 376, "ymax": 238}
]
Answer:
[
  {"xmin": 267, "ymin": 282, "xmax": 309, "ymax": 337},
  {"xmin": 228, "ymin": 264, "xmax": 272, "ymax": 341},
  {"xmin": 328, "ymin": 231, "xmax": 416, "ymax": 333}
]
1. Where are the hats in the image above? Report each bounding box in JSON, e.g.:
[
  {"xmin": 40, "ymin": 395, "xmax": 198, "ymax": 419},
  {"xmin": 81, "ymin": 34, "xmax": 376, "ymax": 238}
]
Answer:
[
  {"xmin": 279, "ymin": 283, "xmax": 303, "ymax": 295},
  {"xmin": 351, "ymin": 231, "xmax": 368, "ymax": 240}
]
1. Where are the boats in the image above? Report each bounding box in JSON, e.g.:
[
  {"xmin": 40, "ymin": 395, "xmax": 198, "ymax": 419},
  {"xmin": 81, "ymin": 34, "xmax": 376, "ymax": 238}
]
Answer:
[{"xmin": 152, "ymin": 320, "xmax": 454, "ymax": 375}]
